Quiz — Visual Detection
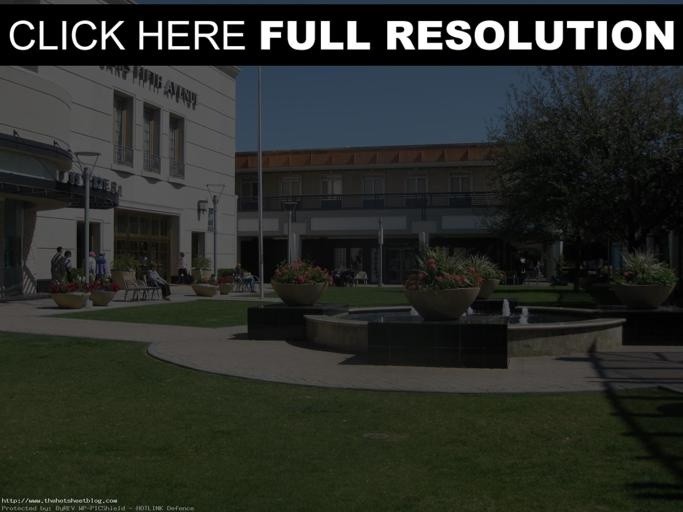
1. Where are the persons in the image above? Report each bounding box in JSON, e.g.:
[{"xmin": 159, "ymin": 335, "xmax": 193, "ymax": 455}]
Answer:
[
  {"xmin": 322, "ymin": 265, "xmax": 355, "ymax": 288},
  {"xmin": 96, "ymin": 251, "xmax": 109, "ymax": 275},
  {"xmin": 175, "ymin": 252, "xmax": 190, "ymax": 284},
  {"xmin": 234, "ymin": 263, "xmax": 256, "ymax": 294},
  {"xmin": 51, "ymin": 246, "xmax": 73, "ymax": 283},
  {"xmin": 147, "ymin": 262, "xmax": 172, "ymax": 301},
  {"xmin": 89, "ymin": 251, "xmax": 96, "ymax": 277}
]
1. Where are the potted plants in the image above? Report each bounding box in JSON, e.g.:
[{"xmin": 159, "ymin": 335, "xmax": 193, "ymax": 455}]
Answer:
[
  {"xmin": 191, "ymin": 255, "xmax": 212, "ymax": 284},
  {"xmin": 110, "ymin": 253, "xmax": 141, "ymax": 289}
]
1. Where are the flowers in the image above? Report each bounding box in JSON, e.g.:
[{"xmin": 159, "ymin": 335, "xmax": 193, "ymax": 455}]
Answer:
[
  {"xmin": 195, "ymin": 275, "xmax": 234, "ymax": 284},
  {"xmin": 608, "ymin": 247, "xmax": 680, "ymax": 289},
  {"xmin": 403, "ymin": 246, "xmax": 507, "ymax": 290},
  {"xmin": 81, "ymin": 278, "xmax": 123, "ymax": 292},
  {"xmin": 47, "ymin": 278, "xmax": 89, "ymax": 293},
  {"xmin": 271, "ymin": 256, "xmax": 334, "ymax": 287}
]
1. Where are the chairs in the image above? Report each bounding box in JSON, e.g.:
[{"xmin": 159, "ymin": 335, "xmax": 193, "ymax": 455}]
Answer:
[
  {"xmin": 123, "ymin": 279, "xmax": 161, "ymax": 302},
  {"xmin": 354, "ymin": 271, "xmax": 368, "ymax": 289}
]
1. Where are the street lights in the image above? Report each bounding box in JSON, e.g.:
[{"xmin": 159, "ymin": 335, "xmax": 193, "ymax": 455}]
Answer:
[
  {"xmin": 378, "ymin": 218, "xmax": 386, "ymax": 286},
  {"xmin": 205, "ymin": 184, "xmax": 222, "ymax": 285},
  {"xmin": 283, "ymin": 200, "xmax": 296, "ymax": 264},
  {"xmin": 75, "ymin": 151, "xmax": 99, "ymax": 288}
]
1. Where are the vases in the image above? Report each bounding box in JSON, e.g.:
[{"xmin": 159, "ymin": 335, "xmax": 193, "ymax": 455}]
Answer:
[
  {"xmin": 272, "ymin": 280, "xmax": 327, "ymax": 306},
  {"xmin": 594, "ymin": 284, "xmax": 624, "ymax": 305},
  {"xmin": 610, "ymin": 282, "xmax": 677, "ymax": 309},
  {"xmin": 191, "ymin": 284, "xmax": 219, "ymax": 297},
  {"xmin": 477, "ymin": 279, "xmax": 501, "ymax": 299},
  {"xmin": 51, "ymin": 293, "xmax": 91, "ymax": 309},
  {"xmin": 89, "ymin": 291, "xmax": 117, "ymax": 306},
  {"xmin": 216, "ymin": 283, "xmax": 236, "ymax": 296},
  {"xmin": 404, "ymin": 287, "xmax": 481, "ymax": 322}
]
